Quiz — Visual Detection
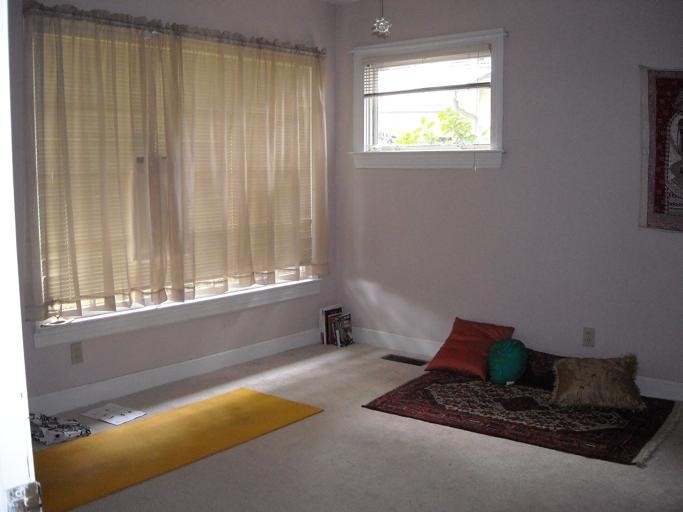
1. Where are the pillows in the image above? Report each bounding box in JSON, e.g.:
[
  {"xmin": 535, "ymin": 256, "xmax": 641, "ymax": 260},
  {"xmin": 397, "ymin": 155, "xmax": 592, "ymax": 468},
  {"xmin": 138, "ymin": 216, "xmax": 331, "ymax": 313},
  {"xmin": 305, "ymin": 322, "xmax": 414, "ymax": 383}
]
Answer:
[{"xmin": 422, "ymin": 315, "xmax": 646, "ymax": 414}]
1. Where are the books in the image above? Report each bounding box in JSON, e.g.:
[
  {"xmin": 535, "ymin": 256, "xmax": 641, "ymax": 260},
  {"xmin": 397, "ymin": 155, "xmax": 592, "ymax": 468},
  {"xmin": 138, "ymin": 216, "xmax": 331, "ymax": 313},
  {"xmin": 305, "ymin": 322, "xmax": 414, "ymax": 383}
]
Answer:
[
  {"xmin": 29, "ymin": 412, "xmax": 90, "ymax": 445},
  {"xmin": 317, "ymin": 302, "xmax": 355, "ymax": 346}
]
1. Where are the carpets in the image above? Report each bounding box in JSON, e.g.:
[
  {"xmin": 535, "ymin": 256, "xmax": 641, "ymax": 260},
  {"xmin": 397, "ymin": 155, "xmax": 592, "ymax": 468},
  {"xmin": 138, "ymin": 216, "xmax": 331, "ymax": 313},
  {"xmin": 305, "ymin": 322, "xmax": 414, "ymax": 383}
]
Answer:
[
  {"xmin": 360, "ymin": 369, "xmax": 682, "ymax": 468},
  {"xmin": 32, "ymin": 385, "xmax": 325, "ymax": 512}
]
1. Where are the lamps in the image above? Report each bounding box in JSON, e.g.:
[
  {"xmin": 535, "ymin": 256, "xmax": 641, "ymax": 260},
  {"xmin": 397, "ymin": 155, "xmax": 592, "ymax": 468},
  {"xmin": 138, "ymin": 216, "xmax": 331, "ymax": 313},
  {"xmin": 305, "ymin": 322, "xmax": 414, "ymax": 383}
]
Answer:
[{"xmin": 371, "ymin": 0, "xmax": 393, "ymax": 37}]
